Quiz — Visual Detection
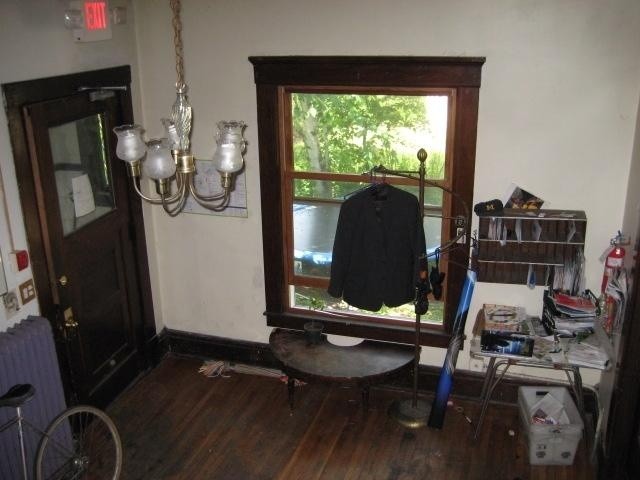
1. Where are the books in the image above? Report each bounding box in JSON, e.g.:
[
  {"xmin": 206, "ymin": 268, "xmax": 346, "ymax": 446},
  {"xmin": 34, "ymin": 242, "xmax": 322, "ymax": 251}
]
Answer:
[
  {"xmin": 552, "ymin": 290, "xmax": 597, "ymax": 318},
  {"xmin": 480, "ymin": 329, "xmax": 536, "ymax": 359}
]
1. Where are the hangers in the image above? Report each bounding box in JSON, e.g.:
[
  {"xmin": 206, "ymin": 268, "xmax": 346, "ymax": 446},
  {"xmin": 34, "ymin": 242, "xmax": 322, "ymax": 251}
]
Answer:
[
  {"xmin": 440, "ymin": 223, "xmax": 479, "ymax": 273},
  {"xmin": 343, "ymin": 165, "xmax": 392, "ymax": 205}
]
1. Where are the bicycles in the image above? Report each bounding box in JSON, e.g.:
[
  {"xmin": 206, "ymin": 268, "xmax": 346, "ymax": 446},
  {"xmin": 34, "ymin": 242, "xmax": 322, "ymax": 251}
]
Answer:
[{"xmin": 1, "ymin": 379, "xmax": 125, "ymax": 480}]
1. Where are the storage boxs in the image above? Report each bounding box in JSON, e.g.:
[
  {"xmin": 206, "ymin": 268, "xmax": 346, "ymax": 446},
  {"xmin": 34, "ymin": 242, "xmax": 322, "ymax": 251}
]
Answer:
[{"xmin": 517, "ymin": 385, "xmax": 586, "ymax": 467}]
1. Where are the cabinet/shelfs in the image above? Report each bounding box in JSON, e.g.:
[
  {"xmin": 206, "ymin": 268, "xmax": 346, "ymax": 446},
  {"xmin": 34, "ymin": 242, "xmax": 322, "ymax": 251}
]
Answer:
[{"xmin": 477, "ymin": 207, "xmax": 587, "ymax": 286}]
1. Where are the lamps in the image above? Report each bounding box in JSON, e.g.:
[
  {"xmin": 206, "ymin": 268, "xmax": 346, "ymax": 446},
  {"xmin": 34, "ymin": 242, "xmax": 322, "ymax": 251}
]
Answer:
[{"xmin": 112, "ymin": 0, "xmax": 247, "ymax": 217}]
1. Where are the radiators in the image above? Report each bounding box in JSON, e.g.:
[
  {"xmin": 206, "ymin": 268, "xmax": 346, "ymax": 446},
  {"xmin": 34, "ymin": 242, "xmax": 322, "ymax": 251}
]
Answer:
[{"xmin": 0, "ymin": 315, "xmax": 75, "ymax": 480}]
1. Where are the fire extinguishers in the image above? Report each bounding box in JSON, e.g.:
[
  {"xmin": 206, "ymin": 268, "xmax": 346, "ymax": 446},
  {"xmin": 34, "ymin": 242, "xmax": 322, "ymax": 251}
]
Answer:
[{"xmin": 602, "ymin": 230, "xmax": 631, "ymax": 296}]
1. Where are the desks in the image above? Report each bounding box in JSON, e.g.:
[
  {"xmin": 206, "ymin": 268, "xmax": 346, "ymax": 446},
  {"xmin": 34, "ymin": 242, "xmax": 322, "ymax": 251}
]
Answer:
[{"xmin": 470, "ymin": 320, "xmax": 608, "ymax": 449}]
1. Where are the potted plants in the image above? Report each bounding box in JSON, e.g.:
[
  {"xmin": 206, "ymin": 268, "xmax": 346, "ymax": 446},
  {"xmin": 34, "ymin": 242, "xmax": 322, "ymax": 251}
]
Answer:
[{"xmin": 298, "ymin": 295, "xmax": 327, "ymax": 345}]
